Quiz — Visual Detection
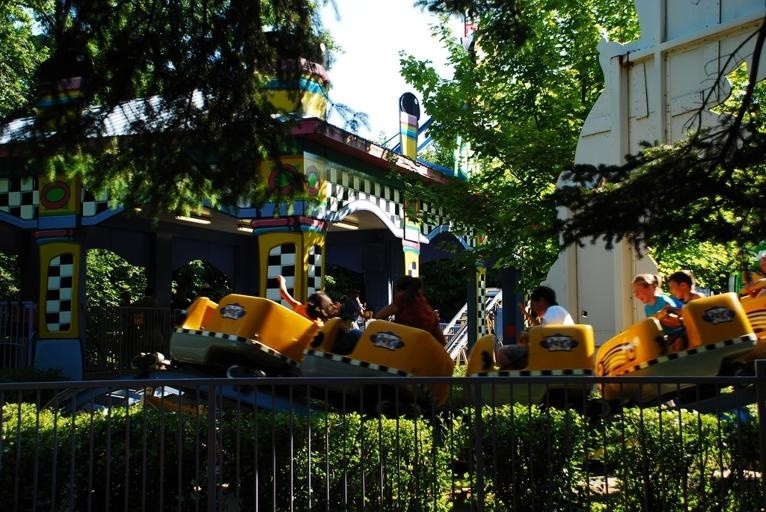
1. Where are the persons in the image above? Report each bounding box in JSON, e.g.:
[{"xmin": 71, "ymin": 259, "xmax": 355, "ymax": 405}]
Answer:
[
  {"xmin": 274, "ymin": 254, "xmax": 578, "ymax": 369},
  {"xmin": 741, "ymin": 268, "xmax": 765, "ymax": 299},
  {"xmin": 651, "ymin": 271, "xmax": 701, "ymax": 352},
  {"xmin": 630, "ymin": 271, "xmax": 685, "ymax": 354},
  {"xmin": 738, "ymin": 256, "xmax": 766, "ymax": 302},
  {"xmin": 117, "ymin": 280, "xmax": 226, "ymax": 369}
]
